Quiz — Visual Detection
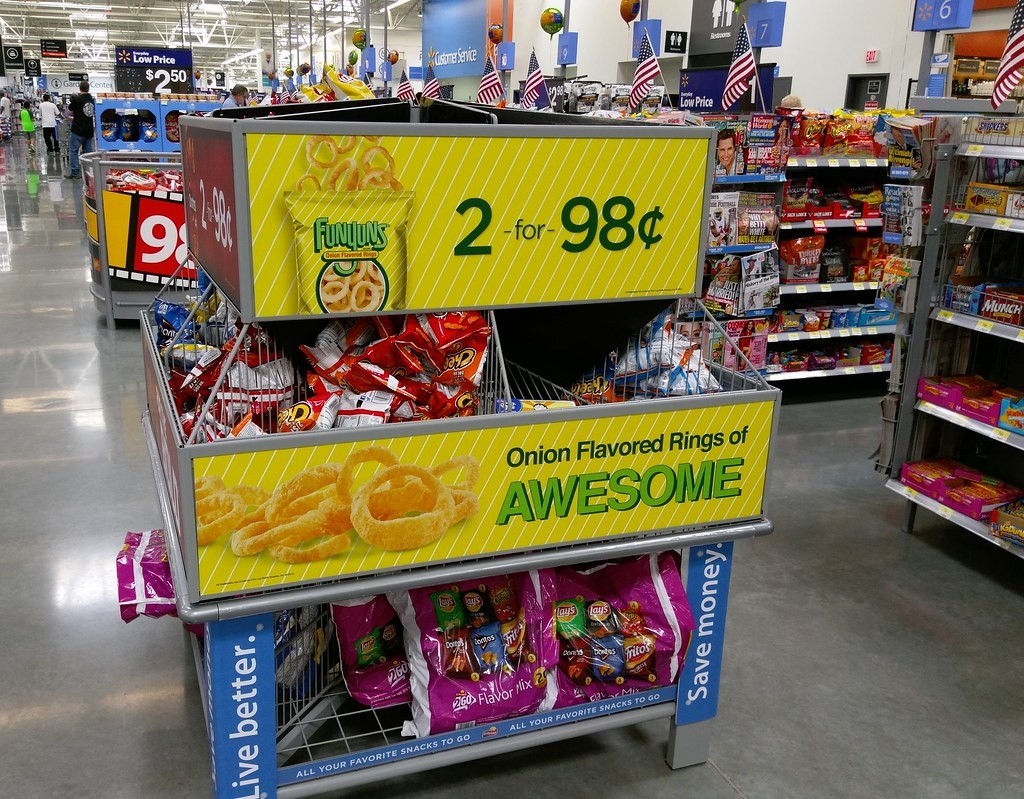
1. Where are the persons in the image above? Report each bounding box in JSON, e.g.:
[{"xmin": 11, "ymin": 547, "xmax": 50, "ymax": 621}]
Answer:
[
  {"xmin": 710, "ymin": 208, "xmax": 736, "ymax": 245},
  {"xmin": 780, "ymin": 94, "xmax": 802, "ymax": 107},
  {"xmin": 767, "ymin": 216, "xmax": 779, "ymax": 232},
  {"xmin": 222, "ymin": 84, "xmax": 248, "ymax": 109},
  {"xmin": 717, "ymin": 129, "xmax": 737, "ymax": 176},
  {"xmin": 731, "ymin": 321, "xmax": 755, "ymax": 371},
  {"xmin": 0, "ymin": 81, "xmax": 95, "ymax": 179},
  {"xmin": 24, "ymin": 154, "xmax": 84, "ymax": 221},
  {"xmin": 890, "ymin": 126, "xmax": 917, "ymax": 167},
  {"xmin": 774, "ymin": 118, "xmax": 791, "ymax": 147},
  {"xmin": 710, "ymin": 207, "xmax": 726, "ymax": 246},
  {"xmin": 739, "ymin": 215, "xmax": 748, "ymax": 235},
  {"xmin": 680, "ymin": 325, "xmax": 700, "ymax": 348}
]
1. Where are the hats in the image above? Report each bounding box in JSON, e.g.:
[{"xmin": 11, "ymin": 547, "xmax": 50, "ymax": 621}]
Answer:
[{"xmin": 782, "ymin": 95, "xmax": 801, "ymax": 107}]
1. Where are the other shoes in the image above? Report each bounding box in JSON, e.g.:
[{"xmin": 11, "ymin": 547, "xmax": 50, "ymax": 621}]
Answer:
[
  {"xmin": 48, "ymin": 150, "xmax": 54, "ymax": 154},
  {"xmin": 30, "ymin": 144, "xmax": 35, "ymax": 152},
  {"xmin": 64, "ymin": 175, "xmax": 80, "ymax": 179},
  {"xmin": 28, "ymin": 149, "xmax": 32, "ymax": 153},
  {"xmin": 56, "ymin": 152, "xmax": 60, "ymax": 155}
]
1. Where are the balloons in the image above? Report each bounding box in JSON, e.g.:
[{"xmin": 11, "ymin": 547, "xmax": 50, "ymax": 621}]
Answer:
[
  {"xmin": 487, "ymin": 0, "xmax": 640, "ymax": 44},
  {"xmin": 193, "ymin": 29, "xmax": 398, "ymax": 86}
]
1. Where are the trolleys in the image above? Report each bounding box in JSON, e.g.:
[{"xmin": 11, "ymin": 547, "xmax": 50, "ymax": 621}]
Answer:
[{"xmin": 60, "ymin": 120, "xmax": 96, "ymax": 161}]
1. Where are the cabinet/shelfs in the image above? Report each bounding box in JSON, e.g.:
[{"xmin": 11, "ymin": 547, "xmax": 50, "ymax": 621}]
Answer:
[{"xmin": 72, "ymin": 0, "xmax": 1024, "ymax": 799}]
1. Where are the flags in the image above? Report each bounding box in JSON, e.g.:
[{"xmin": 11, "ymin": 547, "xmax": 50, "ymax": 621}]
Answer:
[
  {"xmin": 476, "ymin": 58, "xmax": 503, "ymax": 105},
  {"xmin": 524, "ymin": 52, "xmax": 543, "ymax": 109},
  {"xmin": 991, "ymin": 1, "xmax": 1024, "ymax": 110},
  {"xmin": 722, "ymin": 26, "xmax": 756, "ymax": 111},
  {"xmin": 396, "ymin": 65, "xmax": 440, "ymax": 101},
  {"xmin": 628, "ymin": 36, "xmax": 660, "ymax": 110}
]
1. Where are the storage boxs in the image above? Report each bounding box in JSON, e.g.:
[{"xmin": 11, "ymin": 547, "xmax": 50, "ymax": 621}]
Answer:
[
  {"xmin": 944, "ymin": 274, "xmax": 1009, "ymax": 316},
  {"xmin": 988, "ymin": 498, "xmax": 1024, "ymax": 548},
  {"xmin": 900, "ymin": 456, "xmax": 984, "ymax": 500},
  {"xmin": 998, "ymin": 398, "xmax": 1024, "ymax": 436},
  {"xmin": 964, "ymin": 182, "xmax": 1024, "ymax": 216},
  {"xmin": 1005, "ymin": 193, "xmax": 1024, "ymax": 219},
  {"xmin": 954, "ymin": 385, "xmax": 1024, "ymax": 427},
  {"xmin": 917, "ymin": 372, "xmax": 1001, "ymax": 410},
  {"xmin": 936, "ymin": 476, "xmax": 1024, "ymax": 520},
  {"xmin": 979, "ymin": 283, "xmax": 1024, "ymax": 326}
]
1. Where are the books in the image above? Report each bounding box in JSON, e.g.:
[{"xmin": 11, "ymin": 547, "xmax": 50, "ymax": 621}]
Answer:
[
  {"xmin": 879, "ymin": 113, "xmax": 941, "ymax": 314},
  {"xmin": 678, "ymin": 114, "xmax": 790, "ymax": 373}
]
